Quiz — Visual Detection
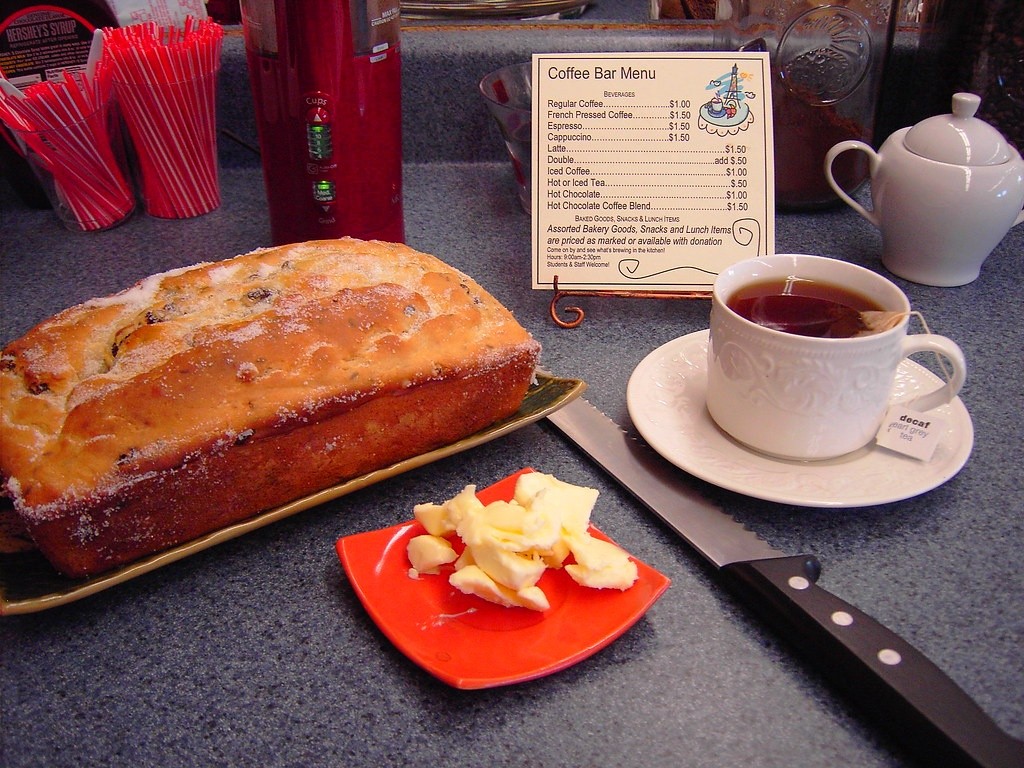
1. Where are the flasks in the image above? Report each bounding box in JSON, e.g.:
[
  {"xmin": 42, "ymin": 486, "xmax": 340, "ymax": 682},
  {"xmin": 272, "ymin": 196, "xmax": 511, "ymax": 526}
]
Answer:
[{"xmin": 240, "ymin": 0, "xmax": 406, "ymax": 246}]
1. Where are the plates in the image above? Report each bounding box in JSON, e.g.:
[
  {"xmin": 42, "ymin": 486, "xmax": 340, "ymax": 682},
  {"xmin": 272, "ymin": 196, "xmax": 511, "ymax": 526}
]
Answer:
[
  {"xmin": 337, "ymin": 468, "xmax": 670, "ymax": 689},
  {"xmin": 0, "ymin": 367, "xmax": 587, "ymax": 614},
  {"xmin": 627, "ymin": 328, "xmax": 975, "ymax": 510}
]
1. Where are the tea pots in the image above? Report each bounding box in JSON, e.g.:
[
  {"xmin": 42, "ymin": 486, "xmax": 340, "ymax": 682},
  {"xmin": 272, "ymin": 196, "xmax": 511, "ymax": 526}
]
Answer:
[{"xmin": 823, "ymin": 93, "xmax": 1024, "ymax": 286}]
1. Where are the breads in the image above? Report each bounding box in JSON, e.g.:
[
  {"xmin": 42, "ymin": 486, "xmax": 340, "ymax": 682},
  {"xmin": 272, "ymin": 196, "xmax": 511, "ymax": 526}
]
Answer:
[{"xmin": 0, "ymin": 236, "xmax": 541, "ymax": 581}]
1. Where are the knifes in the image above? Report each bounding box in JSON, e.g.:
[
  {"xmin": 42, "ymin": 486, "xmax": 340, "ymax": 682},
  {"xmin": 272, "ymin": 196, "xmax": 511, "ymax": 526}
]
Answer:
[{"xmin": 538, "ymin": 399, "xmax": 1023, "ymax": 768}]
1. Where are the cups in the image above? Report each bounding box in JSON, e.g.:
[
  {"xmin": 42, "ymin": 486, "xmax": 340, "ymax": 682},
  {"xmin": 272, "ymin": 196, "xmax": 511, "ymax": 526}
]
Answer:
[
  {"xmin": 479, "ymin": 62, "xmax": 532, "ymax": 214},
  {"xmin": 3, "ymin": 91, "xmax": 137, "ymax": 234},
  {"xmin": 710, "ymin": 0, "xmax": 886, "ymax": 211},
  {"xmin": 911, "ymin": 0, "xmax": 1023, "ymax": 156},
  {"xmin": 107, "ymin": 59, "xmax": 225, "ymax": 222},
  {"xmin": 704, "ymin": 253, "xmax": 966, "ymax": 462}
]
